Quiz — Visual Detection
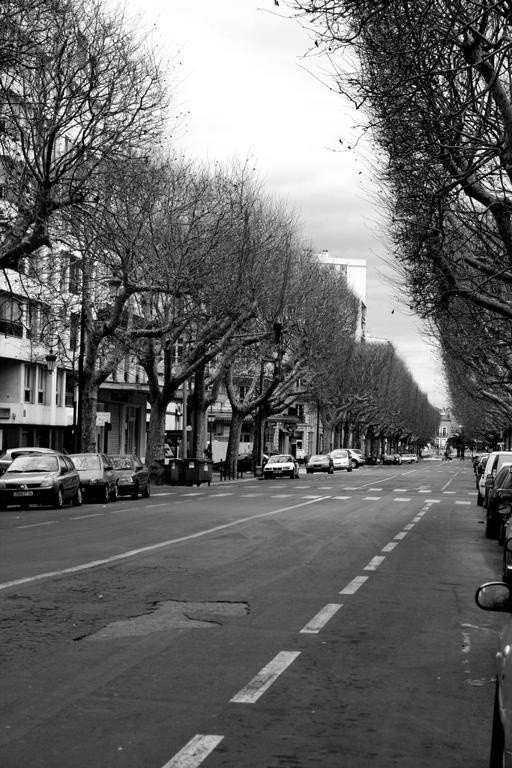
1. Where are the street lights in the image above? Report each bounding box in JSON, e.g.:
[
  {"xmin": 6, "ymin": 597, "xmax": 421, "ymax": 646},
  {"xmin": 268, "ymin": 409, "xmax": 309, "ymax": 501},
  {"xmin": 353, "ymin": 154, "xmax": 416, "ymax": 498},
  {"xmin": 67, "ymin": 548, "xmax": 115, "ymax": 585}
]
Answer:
[{"xmin": 39, "ymin": 318, "xmax": 79, "ymax": 452}]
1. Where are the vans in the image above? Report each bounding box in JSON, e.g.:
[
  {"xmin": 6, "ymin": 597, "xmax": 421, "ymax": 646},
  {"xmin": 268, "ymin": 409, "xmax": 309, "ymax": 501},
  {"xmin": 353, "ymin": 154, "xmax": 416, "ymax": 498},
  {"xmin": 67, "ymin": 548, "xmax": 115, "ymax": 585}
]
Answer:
[{"xmin": 327, "ymin": 447, "xmax": 366, "ymax": 469}]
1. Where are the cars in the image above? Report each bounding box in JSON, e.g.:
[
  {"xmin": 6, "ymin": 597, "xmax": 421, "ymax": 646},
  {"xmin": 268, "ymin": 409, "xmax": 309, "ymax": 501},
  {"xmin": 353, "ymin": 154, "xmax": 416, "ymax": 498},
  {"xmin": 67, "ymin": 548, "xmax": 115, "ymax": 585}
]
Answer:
[
  {"xmin": 305, "ymin": 454, "xmax": 334, "ymax": 474},
  {"xmin": 330, "ymin": 450, "xmax": 353, "ymax": 472},
  {"xmin": 382, "ymin": 453, "xmax": 419, "ymax": 466},
  {"xmin": 1, "ymin": 446, "xmax": 152, "ymax": 510},
  {"xmin": 263, "ymin": 454, "xmax": 299, "ymax": 481},
  {"xmin": 214, "ymin": 453, "xmax": 269, "ymax": 474},
  {"xmin": 472, "ymin": 446, "xmax": 512, "ymax": 766}
]
